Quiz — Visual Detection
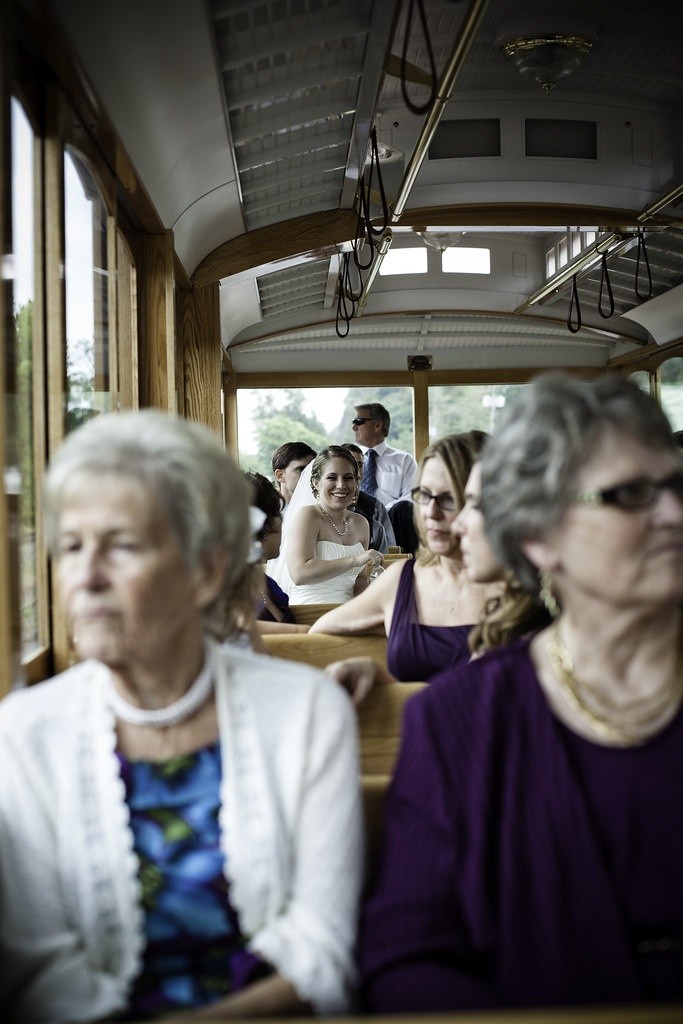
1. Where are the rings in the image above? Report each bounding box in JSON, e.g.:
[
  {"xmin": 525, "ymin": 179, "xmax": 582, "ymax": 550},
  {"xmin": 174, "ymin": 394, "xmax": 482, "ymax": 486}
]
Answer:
[{"xmin": 376, "ymin": 555, "xmax": 378, "ymax": 558}]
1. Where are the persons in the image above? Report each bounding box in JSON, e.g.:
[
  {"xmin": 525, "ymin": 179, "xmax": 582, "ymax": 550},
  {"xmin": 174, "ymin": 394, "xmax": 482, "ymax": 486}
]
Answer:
[
  {"xmin": 353, "ymin": 403, "xmax": 419, "ymax": 555},
  {"xmin": 324, "ymin": 450, "xmax": 560, "ymax": 706},
  {"xmin": 357, "ymin": 367, "xmax": 683, "ymax": 1024},
  {"xmin": 266, "ymin": 445, "xmax": 384, "ymax": 605},
  {"xmin": 272, "ymin": 442, "xmax": 317, "ymax": 517},
  {"xmin": 309, "ymin": 430, "xmax": 510, "ymax": 681},
  {"xmin": 242, "ymin": 472, "xmax": 307, "ymax": 632},
  {"xmin": 341, "ymin": 442, "xmax": 396, "ymax": 556},
  {"xmin": 0, "ymin": 408, "xmax": 361, "ymax": 1023}
]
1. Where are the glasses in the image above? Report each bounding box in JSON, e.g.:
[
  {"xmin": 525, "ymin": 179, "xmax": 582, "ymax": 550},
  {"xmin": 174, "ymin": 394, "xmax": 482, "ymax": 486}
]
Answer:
[
  {"xmin": 560, "ymin": 469, "xmax": 683, "ymax": 511},
  {"xmin": 352, "ymin": 418, "xmax": 375, "ymax": 426},
  {"xmin": 411, "ymin": 485, "xmax": 458, "ymax": 511}
]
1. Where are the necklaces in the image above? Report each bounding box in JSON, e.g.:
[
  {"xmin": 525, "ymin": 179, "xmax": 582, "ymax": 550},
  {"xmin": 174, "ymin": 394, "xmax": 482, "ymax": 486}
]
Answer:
[
  {"xmin": 98, "ymin": 644, "xmax": 214, "ymax": 727},
  {"xmin": 555, "ymin": 622, "xmax": 682, "ymax": 745},
  {"xmin": 319, "ymin": 504, "xmax": 348, "ymax": 536}
]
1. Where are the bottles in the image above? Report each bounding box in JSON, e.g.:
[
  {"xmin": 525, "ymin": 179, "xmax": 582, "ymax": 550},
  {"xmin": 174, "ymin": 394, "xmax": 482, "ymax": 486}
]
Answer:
[{"xmin": 371, "ymin": 564, "xmax": 385, "ymax": 582}]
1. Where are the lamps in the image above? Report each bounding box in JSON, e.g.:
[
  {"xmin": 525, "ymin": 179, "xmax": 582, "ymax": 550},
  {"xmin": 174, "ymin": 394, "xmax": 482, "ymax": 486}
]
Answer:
[{"xmin": 493, "ymin": 24, "xmax": 602, "ymax": 90}]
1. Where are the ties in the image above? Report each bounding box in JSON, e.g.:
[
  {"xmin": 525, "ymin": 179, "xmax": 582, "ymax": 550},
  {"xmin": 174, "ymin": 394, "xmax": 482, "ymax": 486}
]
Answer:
[{"xmin": 360, "ymin": 449, "xmax": 379, "ymax": 496}]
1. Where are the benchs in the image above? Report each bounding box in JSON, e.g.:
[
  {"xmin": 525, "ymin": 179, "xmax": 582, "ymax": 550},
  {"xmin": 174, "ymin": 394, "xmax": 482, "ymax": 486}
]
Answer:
[{"xmin": 261, "ymin": 548, "xmax": 442, "ymax": 820}]
